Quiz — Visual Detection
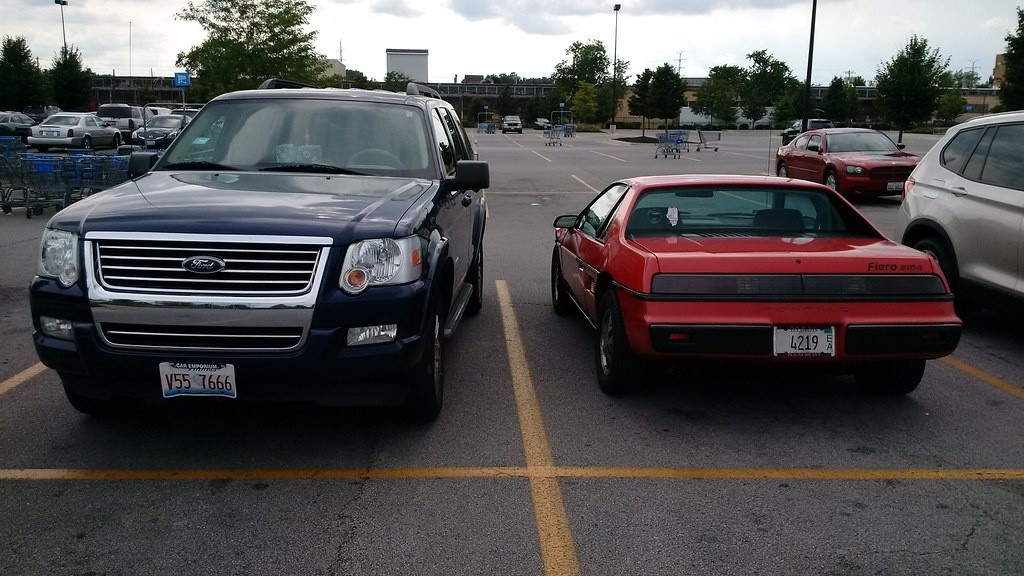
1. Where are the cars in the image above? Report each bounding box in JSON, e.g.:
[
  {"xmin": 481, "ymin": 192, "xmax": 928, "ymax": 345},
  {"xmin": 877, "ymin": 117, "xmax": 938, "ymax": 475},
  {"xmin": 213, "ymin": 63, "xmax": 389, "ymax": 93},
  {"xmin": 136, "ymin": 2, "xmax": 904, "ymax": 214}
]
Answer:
[
  {"xmin": 551, "ymin": 175, "xmax": 963, "ymax": 402},
  {"xmin": 534, "ymin": 117, "xmax": 552, "ymax": 131},
  {"xmin": 775, "ymin": 127, "xmax": 922, "ymax": 205},
  {"xmin": 132, "ymin": 114, "xmax": 194, "ymax": 149},
  {"xmin": 28, "ymin": 112, "xmax": 123, "ymax": 151},
  {"xmin": 0, "ymin": 110, "xmax": 37, "ymax": 136}
]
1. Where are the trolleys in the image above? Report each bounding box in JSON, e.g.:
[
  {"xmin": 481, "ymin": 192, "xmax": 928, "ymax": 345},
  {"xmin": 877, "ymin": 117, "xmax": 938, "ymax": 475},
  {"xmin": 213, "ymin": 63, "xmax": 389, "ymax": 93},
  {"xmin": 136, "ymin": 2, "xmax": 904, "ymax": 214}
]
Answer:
[
  {"xmin": 653, "ymin": 130, "xmax": 691, "ymax": 160},
  {"xmin": 0, "ymin": 137, "xmax": 162, "ymax": 218},
  {"xmin": 566, "ymin": 125, "xmax": 578, "ymax": 139},
  {"xmin": 696, "ymin": 129, "xmax": 722, "ymax": 152},
  {"xmin": 543, "ymin": 127, "xmax": 563, "ymax": 147}
]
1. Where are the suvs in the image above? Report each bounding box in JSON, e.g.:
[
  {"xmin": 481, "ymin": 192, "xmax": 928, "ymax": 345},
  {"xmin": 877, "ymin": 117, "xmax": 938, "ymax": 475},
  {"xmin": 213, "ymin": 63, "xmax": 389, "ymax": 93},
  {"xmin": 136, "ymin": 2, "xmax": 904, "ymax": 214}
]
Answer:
[
  {"xmin": 27, "ymin": 78, "xmax": 492, "ymax": 428},
  {"xmin": 782, "ymin": 119, "xmax": 836, "ymax": 147},
  {"xmin": 501, "ymin": 115, "xmax": 524, "ymax": 134},
  {"xmin": 896, "ymin": 111, "xmax": 1023, "ymax": 316}
]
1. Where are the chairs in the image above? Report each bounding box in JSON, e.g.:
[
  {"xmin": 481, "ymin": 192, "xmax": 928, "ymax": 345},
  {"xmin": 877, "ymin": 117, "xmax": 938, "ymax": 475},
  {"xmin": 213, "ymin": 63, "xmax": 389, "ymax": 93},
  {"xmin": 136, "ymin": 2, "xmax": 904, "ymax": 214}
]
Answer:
[
  {"xmin": 628, "ymin": 206, "xmax": 683, "ymax": 230},
  {"xmin": 752, "ymin": 208, "xmax": 807, "ymax": 231}
]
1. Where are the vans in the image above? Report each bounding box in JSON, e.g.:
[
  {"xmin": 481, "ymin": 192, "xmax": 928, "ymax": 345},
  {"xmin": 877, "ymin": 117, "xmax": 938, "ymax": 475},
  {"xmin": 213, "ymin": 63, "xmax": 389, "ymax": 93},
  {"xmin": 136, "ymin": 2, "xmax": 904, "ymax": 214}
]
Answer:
[
  {"xmin": 147, "ymin": 107, "xmax": 171, "ymax": 117},
  {"xmin": 95, "ymin": 103, "xmax": 146, "ymax": 144},
  {"xmin": 170, "ymin": 108, "xmax": 198, "ymax": 118}
]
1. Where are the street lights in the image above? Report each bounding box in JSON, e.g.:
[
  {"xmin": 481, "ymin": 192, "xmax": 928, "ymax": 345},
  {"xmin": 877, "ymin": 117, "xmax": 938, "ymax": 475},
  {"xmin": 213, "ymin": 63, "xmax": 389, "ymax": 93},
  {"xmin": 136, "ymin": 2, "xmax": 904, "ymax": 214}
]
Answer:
[
  {"xmin": 55, "ymin": 0, "xmax": 71, "ymax": 59},
  {"xmin": 610, "ymin": 3, "xmax": 622, "ymax": 133}
]
